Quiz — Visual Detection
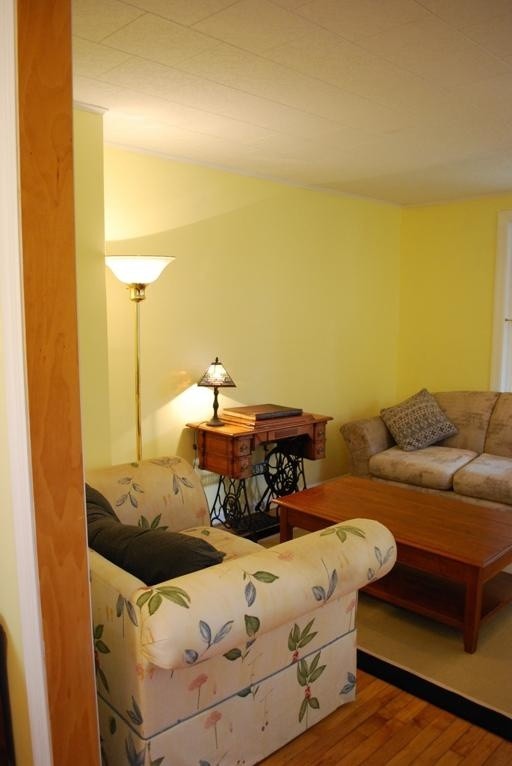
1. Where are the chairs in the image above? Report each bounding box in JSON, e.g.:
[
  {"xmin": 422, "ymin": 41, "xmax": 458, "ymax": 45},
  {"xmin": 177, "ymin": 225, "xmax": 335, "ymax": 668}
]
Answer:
[{"xmin": 85, "ymin": 455, "xmax": 400, "ymax": 766}]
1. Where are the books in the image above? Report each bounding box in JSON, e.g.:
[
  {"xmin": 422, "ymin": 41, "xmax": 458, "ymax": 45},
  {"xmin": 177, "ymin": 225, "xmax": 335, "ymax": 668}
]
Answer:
[{"xmin": 218, "ymin": 404, "xmax": 314, "ymax": 429}]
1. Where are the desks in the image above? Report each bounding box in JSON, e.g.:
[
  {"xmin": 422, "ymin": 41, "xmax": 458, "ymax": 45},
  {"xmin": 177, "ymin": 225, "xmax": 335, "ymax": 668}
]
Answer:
[{"xmin": 185, "ymin": 414, "xmax": 334, "ymax": 541}]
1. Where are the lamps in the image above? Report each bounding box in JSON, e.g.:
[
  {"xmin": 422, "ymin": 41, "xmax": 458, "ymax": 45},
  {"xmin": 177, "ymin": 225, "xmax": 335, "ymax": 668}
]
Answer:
[
  {"xmin": 104, "ymin": 254, "xmax": 176, "ymax": 461},
  {"xmin": 198, "ymin": 356, "xmax": 237, "ymax": 427}
]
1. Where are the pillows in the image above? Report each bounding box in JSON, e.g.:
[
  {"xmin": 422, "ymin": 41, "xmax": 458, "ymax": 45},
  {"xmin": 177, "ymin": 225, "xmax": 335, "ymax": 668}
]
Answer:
[
  {"xmin": 85, "ymin": 483, "xmax": 226, "ymax": 587},
  {"xmin": 381, "ymin": 388, "xmax": 458, "ymax": 452}
]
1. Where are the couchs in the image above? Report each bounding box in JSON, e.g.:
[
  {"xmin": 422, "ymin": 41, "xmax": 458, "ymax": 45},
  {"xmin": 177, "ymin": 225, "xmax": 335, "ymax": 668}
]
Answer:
[{"xmin": 339, "ymin": 390, "xmax": 512, "ymax": 511}]
